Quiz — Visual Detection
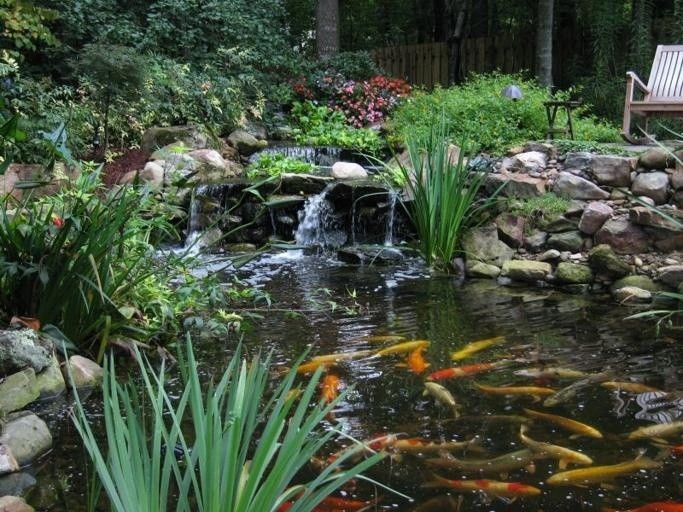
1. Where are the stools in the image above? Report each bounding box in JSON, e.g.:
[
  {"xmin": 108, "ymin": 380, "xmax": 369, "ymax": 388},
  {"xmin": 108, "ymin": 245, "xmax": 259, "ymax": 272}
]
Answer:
[{"xmin": 541, "ymin": 101, "xmax": 582, "ymax": 139}]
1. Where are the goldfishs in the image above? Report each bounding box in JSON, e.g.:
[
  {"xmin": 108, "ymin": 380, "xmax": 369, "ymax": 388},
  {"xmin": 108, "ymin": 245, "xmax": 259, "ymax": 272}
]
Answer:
[{"xmin": 277, "ymin": 335, "xmax": 683, "ymax": 512}]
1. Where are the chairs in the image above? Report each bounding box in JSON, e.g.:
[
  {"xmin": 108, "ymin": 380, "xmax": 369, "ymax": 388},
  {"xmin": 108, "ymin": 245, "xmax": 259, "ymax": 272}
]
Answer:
[{"xmin": 620, "ymin": 44, "xmax": 683, "ymax": 145}]
